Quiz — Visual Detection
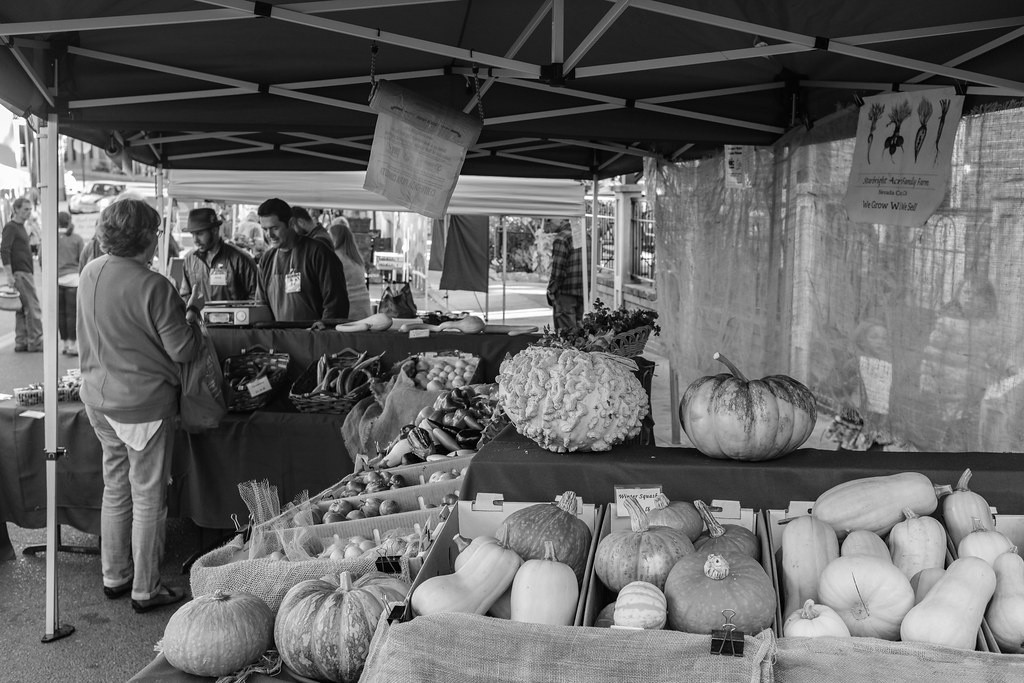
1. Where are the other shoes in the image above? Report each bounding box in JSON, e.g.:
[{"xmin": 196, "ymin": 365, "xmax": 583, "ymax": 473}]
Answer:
[
  {"xmin": 15, "ymin": 345, "xmax": 27, "ymax": 352},
  {"xmin": 61, "ymin": 341, "xmax": 78, "ymax": 355},
  {"xmin": 105, "ymin": 578, "xmax": 133, "ymax": 598},
  {"xmin": 28, "ymin": 339, "xmax": 43, "ymax": 353},
  {"xmin": 132, "ymin": 583, "xmax": 184, "ymax": 613}
]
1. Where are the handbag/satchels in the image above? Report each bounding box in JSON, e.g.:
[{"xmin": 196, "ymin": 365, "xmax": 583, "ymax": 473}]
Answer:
[
  {"xmin": 179, "ymin": 305, "xmax": 227, "ymax": 433},
  {"xmin": 0, "ymin": 284, "xmax": 23, "ymax": 312},
  {"xmin": 377, "ymin": 278, "xmax": 419, "ymax": 317}
]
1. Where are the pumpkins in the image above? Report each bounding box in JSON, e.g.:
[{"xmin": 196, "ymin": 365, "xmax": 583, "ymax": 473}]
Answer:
[
  {"xmin": 677, "ymin": 351, "xmax": 816, "ymax": 462},
  {"xmin": 161, "ymin": 590, "xmax": 276, "ymax": 678},
  {"xmin": 274, "ymin": 571, "xmax": 410, "ymax": 683},
  {"xmin": 407, "ymin": 489, "xmax": 776, "ymax": 635},
  {"xmin": 497, "ymin": 343, "xmax": 649, "ymax": 454},
  {"xmin": 775, "ymin": 467, "xmax": 1024, "ymax": 656},
  {"xmin": 334, "ymin": 313, "xmax": 540, "ymax": 337}
]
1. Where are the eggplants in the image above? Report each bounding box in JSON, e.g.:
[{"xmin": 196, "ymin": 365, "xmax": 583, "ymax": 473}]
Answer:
[{"xmin": 370, "ymin": 388, "xmax": 495, "ymax": 466}]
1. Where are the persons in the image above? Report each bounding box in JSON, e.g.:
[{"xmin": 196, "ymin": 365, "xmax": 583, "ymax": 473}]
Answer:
[
  {"xmin": 914, "ymin": 273, "xmax": 1020, "ymax": 452},
  {"xmin": 75, "ymin": 199, "xmax": 205, "ymax": 613},
  {"xmin": 163, "ymin": 198, "xmax": 373, "ymax": 323},
  {"xmin": 0, "ymin": 198, "xmax": 43, "ymax": 352},
  {"xmin": 823, "ymin": 317, "xmax": 894, "ymax": 451},
  {"xmin": 78, "ymin": 193, "xmax": 144, "ymax": 277},
  {"xmin": 547, "ymin": 217, "xmax": 586, "ymax": 330},
  {"xmin": 37, "ymin": 212, "xmax": 85, "ymax": 356}
]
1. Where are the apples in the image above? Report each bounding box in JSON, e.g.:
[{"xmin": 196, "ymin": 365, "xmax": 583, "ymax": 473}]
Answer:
[{"xmin": 412, "ymin": 358, "xmax": 476, "ymax": 390}]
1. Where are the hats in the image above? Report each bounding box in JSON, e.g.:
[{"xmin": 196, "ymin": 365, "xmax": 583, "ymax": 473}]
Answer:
[{"xmin": 182, "ymin": 209, "xmax": 223, "ymax": 232}]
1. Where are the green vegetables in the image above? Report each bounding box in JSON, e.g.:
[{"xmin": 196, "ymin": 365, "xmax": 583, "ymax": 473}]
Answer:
[{"xmin": 539, "ymin": 298, "xmax": 661, "ymax": 351}]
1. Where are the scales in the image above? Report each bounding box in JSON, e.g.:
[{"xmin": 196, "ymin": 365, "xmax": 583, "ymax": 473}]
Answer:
[{"xmin": 199, "ymin": 299, "xmax": 276, "ymax": 329}]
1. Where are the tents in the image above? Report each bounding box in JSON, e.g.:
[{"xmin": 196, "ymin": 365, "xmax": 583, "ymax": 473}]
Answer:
[{"xmin": 161, "ymin": 170, "xmax": 588, "ymax": 320}]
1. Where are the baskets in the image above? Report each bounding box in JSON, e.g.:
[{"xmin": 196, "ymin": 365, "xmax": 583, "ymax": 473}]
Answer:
[
  {"xmin": 595, "ymin": 324, "xmax": 652, "ymax": 358},
  {"xmin": 288, "ymin": 348, "xmax": 381, "ymax": 414},
  {"xmin": 217, "ymin": 343, "xmax": 290, "ymax": 413},
  {"xmin": 13, "ymin": 369, "xmax": 80, "ymax": 406}
]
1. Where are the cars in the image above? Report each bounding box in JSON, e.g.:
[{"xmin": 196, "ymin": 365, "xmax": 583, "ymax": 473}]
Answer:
[
  {"xmin": 600, "ymin": 211, "xmax": 656, "ymax": 281},
  {"xmin": 69, "ymin": 182, "xmax": 126, "ymax": 214}
]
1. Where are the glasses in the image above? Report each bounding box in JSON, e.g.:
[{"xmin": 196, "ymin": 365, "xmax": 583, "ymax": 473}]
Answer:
[
  {"xmin": 154, "ymin": 229, "xmax": 163, "ymax": 238},
  {"xmin": 547, "ymin": 219, "xmax": 551, "ymax": 222}
]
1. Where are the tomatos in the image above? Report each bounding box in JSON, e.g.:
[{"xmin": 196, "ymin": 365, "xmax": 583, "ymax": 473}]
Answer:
[{"xmin": 292, "ymin": 469, "xmax": 460, "ymax": 560}]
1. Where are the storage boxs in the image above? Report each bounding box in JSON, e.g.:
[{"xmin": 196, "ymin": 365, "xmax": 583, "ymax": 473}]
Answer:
[
  {"xmin": 193, "ymin": 358, "xmax": 1023, "ymax": 661},
  {"xmin": 348, "ymin": 217, "xmax": 406, "ymax": 271}
]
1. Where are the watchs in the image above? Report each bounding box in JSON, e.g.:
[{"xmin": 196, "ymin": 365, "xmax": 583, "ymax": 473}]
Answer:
[{"xmin": 186, "ymin": 305, "xmax": 200, "ymax": 318}]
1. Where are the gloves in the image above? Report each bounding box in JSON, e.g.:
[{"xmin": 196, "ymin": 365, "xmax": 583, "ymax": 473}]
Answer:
[{"xmin": 547, "ymin": 290, "xmax": 557, "ymax": 305}]
